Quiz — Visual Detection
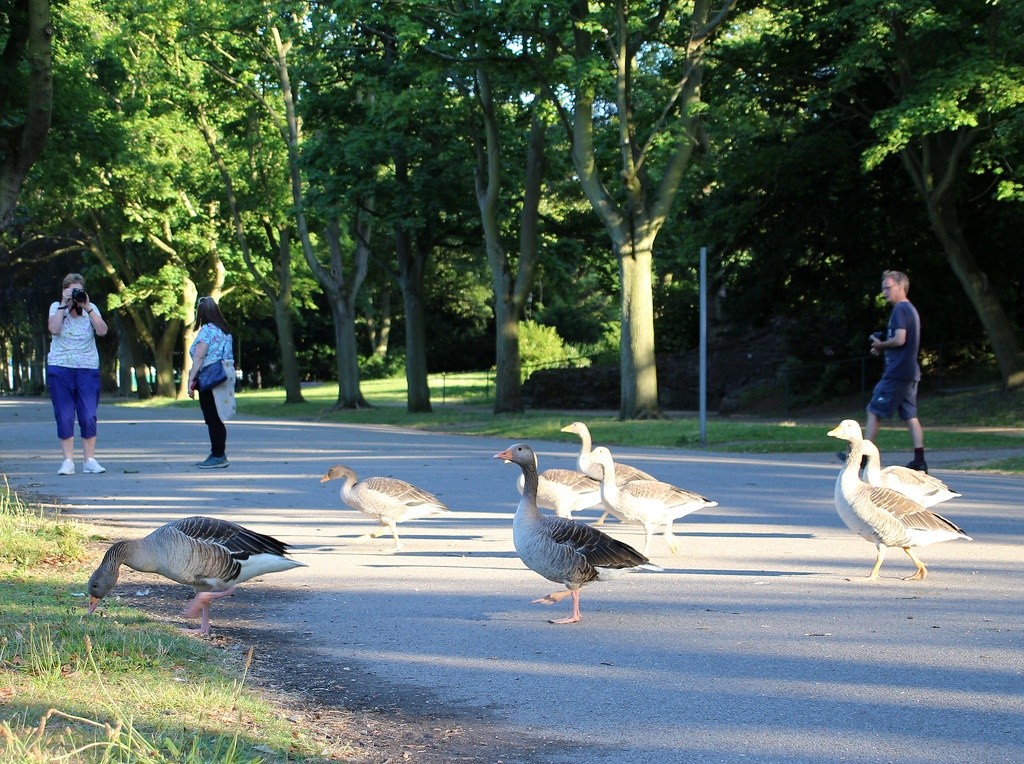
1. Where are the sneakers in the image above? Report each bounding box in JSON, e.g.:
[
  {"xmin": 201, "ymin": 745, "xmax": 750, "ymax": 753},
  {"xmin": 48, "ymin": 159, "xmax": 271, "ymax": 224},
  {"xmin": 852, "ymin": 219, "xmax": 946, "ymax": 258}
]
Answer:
[
  {"xmin": 57, "ymin": 458, "xmax": 76, "ymax": 475},
  {"xmin": 198, "ymin": 453, "xmax": 229, "ymax": 468},
  {"xmin": 82, "ymin": 457, "xmax": 106, "ymax": 473}
]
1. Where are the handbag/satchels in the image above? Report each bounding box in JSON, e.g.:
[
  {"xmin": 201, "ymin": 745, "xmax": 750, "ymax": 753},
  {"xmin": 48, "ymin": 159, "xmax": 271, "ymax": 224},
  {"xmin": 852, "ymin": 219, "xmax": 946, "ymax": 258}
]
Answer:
[{"xmin": 196, "ymin": 345, "xmax": 228, "ymax": 391}]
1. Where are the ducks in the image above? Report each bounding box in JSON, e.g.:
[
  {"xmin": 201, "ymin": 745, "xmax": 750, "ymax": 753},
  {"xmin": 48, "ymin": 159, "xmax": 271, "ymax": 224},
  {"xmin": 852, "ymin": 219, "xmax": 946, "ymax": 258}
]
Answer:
[
  {"xmin": 826, "ymin": 419, "xmax": 974, "ymax": 583},
  {"xmin": 87, "ymin": 516, "xmax": 310, "ymax": 638},
  {"xmin": 492, "ymin": 421, "xmax": 719, "ymax": 625},
  {"xmin": 320, "ymin": 464, "xmax": 449, "ymax": 546}
]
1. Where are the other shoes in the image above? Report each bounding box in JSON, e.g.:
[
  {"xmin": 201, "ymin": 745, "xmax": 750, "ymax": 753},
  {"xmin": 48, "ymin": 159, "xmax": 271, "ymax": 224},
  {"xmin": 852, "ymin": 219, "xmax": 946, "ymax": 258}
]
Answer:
[
  {"xmin": 904, "ymin": 460, "xmax": 929, "ymax": 473},
  {"xmin": 836, "ymin": 451, "xmax": 868, "ymax": 470}
]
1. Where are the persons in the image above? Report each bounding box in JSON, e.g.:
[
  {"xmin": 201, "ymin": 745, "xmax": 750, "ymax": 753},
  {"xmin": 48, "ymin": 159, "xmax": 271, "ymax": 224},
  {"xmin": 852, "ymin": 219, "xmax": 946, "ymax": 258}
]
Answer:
[
  {"xmin": 836, "ymin": 271, "xmax": 927, "ymax": 474},
  {"xmin": 46, "ymin": 274, "xmax": 106, "ymax": 475},
  {"xmin": 187, "ymin": 297, "xmax": 234, "ymax": 470}
]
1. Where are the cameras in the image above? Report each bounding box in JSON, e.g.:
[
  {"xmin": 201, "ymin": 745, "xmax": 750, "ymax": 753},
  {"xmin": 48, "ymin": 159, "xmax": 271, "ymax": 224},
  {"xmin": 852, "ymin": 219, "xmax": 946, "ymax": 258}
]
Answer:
[{"xmin": 71, "ymin": 288, "xmax": 86, "ymax": 302}]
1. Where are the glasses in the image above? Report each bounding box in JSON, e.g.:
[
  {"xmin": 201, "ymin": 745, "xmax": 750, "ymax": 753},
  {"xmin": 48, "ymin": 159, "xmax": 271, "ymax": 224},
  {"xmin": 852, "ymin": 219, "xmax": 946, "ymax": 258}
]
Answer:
[
  {"xmin": 197, "ymin": 296, "xmax": 212, "ymax": 304},
  {"xmin": 882, "ymin": 284, "xmax": 895, "ymax": 291}
]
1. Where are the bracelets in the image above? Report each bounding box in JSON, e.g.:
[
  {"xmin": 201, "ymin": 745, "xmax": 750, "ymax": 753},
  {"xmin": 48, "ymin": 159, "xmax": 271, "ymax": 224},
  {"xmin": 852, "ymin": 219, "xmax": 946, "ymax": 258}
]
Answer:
[{"xmin": 87, "ymin": 309, "xmax": 93, "ymax": 313}]
